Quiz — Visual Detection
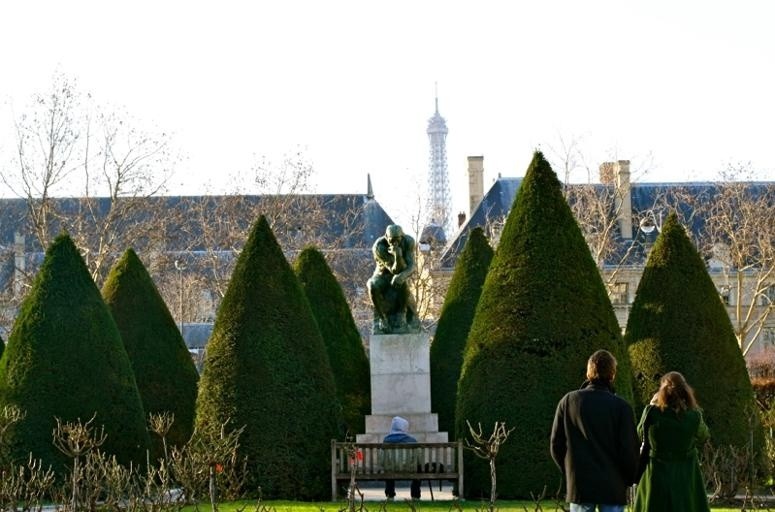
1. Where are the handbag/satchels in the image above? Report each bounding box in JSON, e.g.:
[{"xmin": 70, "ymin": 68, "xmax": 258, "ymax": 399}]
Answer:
[
  {"xmin": 417, "ymin": 463, "xmax": 444, "ymax": 474},
  {"xmin": 633, "ymin": 440, "xmax": 649, "ymax": 484}
]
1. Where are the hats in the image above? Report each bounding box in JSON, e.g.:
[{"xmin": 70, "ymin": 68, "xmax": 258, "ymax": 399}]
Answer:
[{"xmin": 391, "ymin": 416, "xmax": 409, "ymax": 434}]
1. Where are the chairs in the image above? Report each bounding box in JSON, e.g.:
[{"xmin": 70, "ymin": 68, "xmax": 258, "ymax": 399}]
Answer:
[{"xmin": 330, "ymin": 438, "xmax": 465, "ymax": 502}]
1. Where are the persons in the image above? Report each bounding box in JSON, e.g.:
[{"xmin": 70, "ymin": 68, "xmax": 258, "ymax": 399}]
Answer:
[
  {"xmin": 637, "ymin": 371, "xmax": 711, "ymax": 512},
  {"xmin": 382, "ymin": 417, "xmax": 422, "ymax": 502},
  {"xmin": 367, "ymin": 224, "xmax": 419, "ymax": 334},
  {"xmin": 549, "ymin": 349, "xmax": 641, "ymax": 512}
]
